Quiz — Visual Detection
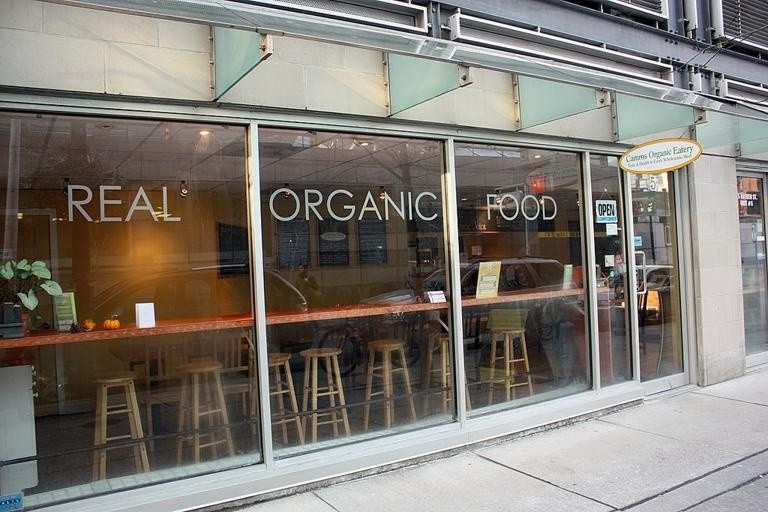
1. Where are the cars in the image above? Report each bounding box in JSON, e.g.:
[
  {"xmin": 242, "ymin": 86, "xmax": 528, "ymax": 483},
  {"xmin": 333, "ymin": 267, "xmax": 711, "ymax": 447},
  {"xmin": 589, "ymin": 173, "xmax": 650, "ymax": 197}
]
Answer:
[
  {"xmin": 0, "ymin": 275, "xmax": 27, "ymax": 339},
  {"xmin": 577, "ymin": 265, "xmax": 673, "ymax": 328}
]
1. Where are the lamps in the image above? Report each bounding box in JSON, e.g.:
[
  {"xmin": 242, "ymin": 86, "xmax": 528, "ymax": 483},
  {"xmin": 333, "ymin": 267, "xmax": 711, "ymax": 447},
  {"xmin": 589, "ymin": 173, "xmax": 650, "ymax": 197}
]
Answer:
[
  {"xmin": 283, "ymin": 183, "xmax": 290, "ymax": 198},
  {"xmin": 62, "ymin": 178, "xmax": 71, "ymax": 196},
  {"xmin": 378, "ymin": 186, "xmax": 385, "ymax": 199},
  {"xmin": 179, "ymin": 181, "xmax": 188, "ymax": 197}
]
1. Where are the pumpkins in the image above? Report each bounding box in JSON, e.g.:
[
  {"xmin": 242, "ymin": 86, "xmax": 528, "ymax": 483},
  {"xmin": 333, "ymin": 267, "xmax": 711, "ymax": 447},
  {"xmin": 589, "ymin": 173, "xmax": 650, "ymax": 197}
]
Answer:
[
  {"xmin": 102, "ymin": 315, "xmax": 120, "ymax": 330},
  {"xmin": 83, "ymin": 319, "xmax": 97, "ymax": 331}
]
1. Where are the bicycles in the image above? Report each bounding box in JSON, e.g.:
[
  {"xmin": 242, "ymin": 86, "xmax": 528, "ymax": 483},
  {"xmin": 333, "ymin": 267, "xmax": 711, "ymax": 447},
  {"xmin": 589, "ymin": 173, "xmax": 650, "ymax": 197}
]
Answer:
[{"xmin": 318, "ymin": 314, "xmax": 421, "ymax": 375}]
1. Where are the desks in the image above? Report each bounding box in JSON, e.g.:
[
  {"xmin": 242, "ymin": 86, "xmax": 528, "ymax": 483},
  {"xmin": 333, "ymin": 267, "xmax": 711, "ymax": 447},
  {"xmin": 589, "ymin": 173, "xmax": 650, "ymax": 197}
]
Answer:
[{"xmin": 0, "ymin": 287, "xmax": 609, "ymax": 349}]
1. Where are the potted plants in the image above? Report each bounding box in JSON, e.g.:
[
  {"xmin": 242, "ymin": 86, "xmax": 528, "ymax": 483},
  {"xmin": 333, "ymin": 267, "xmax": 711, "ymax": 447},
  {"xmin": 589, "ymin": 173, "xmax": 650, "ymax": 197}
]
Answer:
[{"xmin": 0, "ymin": 259, "xmax": 63, "ymax": 338}]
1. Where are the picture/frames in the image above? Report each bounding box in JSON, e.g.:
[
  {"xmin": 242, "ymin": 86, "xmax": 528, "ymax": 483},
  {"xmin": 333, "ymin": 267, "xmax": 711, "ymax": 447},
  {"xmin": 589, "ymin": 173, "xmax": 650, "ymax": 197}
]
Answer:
[{"xmin": 217, "ymin": 217, "xmax": 387, "ymax": 278}]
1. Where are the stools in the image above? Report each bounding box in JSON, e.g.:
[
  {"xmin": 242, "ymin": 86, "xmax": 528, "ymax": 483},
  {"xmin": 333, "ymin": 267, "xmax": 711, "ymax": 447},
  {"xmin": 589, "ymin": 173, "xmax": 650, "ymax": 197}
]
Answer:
[
  {"xmin": 88, "ymin": 370, "xmax": 150, "ymax": 481},
  {"xmin": 300, "ymin": 347, "xmax": 351, "ymax": 443},
  {"xmin": 423, "ymin": 332, "xmax": 472, "ymax": 418},
  {"xmin": 486, "ymin": 327, "xmax": 533, "ymax": 405},
  {"xmin": 361, "ymin": 340, "xmax": 416, "ymax": 433},
  {"xmin": 251, "ymin": 352, "xmax": 305, "ymax": 450},
  {"xmin": 177, "ymin": 362, "xmax": 236, "ymax": 466}
]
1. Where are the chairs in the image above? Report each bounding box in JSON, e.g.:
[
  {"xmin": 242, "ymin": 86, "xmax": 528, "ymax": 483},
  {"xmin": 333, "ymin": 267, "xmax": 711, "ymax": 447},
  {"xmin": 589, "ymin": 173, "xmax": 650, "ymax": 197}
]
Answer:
[
  {"xmin": 624, "ymin": 290, "xmax": 649, "ymax": 355},
  {"xmin": 145, "ymin": 330, "xmax": 253, "ymax": 453}
]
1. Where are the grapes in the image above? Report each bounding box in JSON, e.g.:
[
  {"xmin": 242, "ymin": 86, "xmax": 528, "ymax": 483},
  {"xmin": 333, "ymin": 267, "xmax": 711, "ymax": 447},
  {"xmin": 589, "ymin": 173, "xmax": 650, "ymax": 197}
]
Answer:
[{"xmin": 71, "ymin": 324, "xmax": 81, "ymax": 333}]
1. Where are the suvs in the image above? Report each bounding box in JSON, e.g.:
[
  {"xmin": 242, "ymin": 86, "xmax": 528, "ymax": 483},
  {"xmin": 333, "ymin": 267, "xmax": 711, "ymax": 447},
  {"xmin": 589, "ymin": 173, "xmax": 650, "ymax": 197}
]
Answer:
[
  {"xmin": 358, "ymin": 257, "xmax": 578, "ymax": 356},
  {"xmin": 7, "ymin": 262, "xmax": 316, "ymax": 412}
]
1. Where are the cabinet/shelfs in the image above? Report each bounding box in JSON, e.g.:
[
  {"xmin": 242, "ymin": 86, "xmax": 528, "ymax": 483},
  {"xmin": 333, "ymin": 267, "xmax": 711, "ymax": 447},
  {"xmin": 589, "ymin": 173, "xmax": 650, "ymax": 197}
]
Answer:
[{"xmin": 0, "ymin": 364, "xmax": 38, "ymax": 497}]
